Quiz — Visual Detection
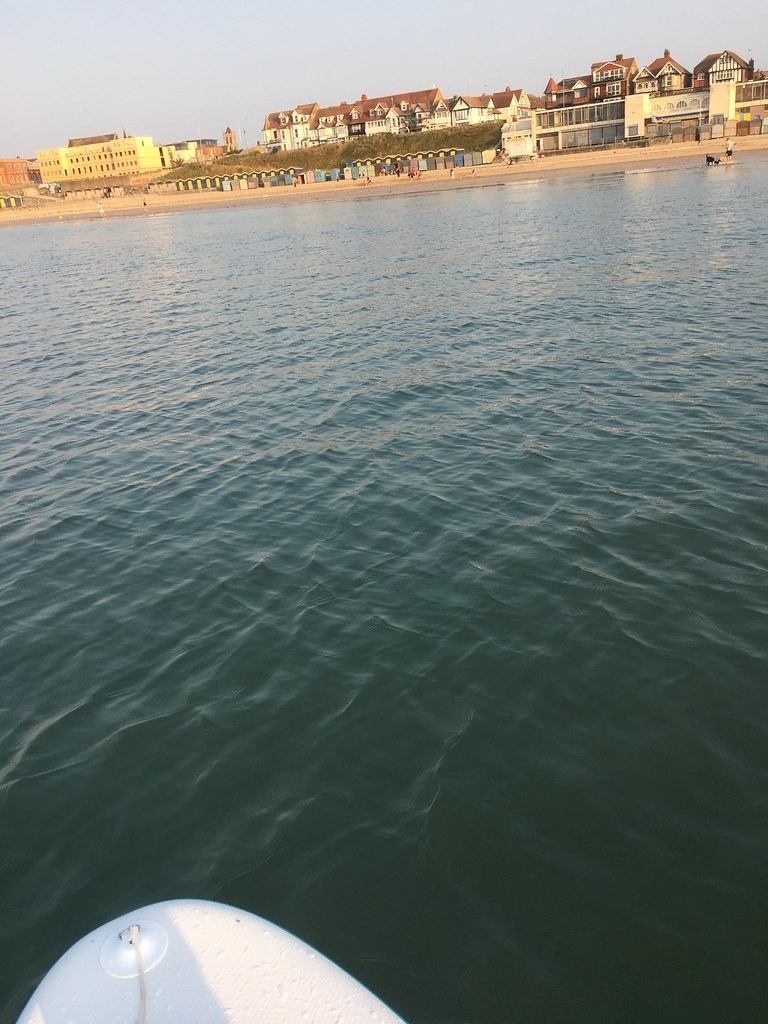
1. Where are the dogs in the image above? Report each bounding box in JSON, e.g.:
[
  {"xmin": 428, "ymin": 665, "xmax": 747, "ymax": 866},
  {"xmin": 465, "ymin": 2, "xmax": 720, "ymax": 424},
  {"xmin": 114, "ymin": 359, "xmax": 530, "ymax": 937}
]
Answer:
[{"xmin": 706, "ymin": 154, "xmax": 728, "ymax": 166}]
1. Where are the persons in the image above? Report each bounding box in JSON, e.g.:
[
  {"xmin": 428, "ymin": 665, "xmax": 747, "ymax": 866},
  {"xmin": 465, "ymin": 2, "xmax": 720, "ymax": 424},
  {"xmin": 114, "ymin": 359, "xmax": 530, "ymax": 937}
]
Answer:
[
  {"xmin": 408, "ymin": 167, "xmax": 422, "ymax": 179},
  {"xmin": 450, "ymin": 170, "xmax": 455, "ymax": 178},
  {"xmin": 142, "ymin": 202, "xmax": 148, "ymax": 212},
  {"xmin": 471, "ymin": 170, "xmax": 477, "ymax": 176},
  {"xmin": 361, "ymin": 172, "xmax": 371, "ymax": 186},
  {"xmin": 133, "ymin": 187, "xmax": 134, "ymax": 195},
  {"xmin": 98, "ymin": 203, "xmax": 106, "ymax": 217},
  {"xmin": 726, "ymin": 138, "xmax": 733, "ymax": 163},
  {"xmin": 393, "ymin": 165, "xmax": 400, "ymax": 177},
  {"xmin": 293, "ymin": 180, "xmax": 296, "ymax": 190},
  {"xmin": 697, "ymin": 132, "xmax": 700, "ymax": 146},
  {"xmin": 498, "ymin": 150, "xmax": 513, "ymax": 169}
]
